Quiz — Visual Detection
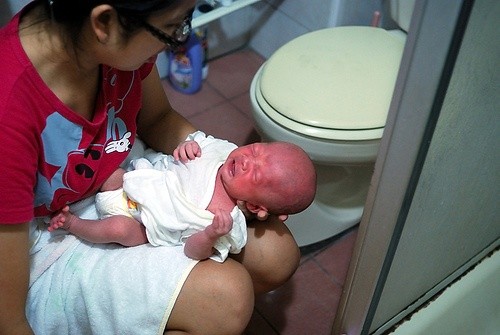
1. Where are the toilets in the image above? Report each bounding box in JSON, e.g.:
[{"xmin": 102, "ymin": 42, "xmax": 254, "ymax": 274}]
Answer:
[{"xmin": 248, "ymin": 0, "xmax": 418, "ymax": 247}]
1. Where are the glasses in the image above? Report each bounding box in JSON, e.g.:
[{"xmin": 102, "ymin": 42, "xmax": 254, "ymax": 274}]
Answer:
[{"xmin": 114, "ymin": 6, "xmax": 192, "ymax": 46}]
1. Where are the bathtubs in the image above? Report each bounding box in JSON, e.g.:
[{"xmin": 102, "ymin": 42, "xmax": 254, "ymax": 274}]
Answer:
[{"xmin": 154, "ymin": 0, "xmax": 275, "ymax": 80}]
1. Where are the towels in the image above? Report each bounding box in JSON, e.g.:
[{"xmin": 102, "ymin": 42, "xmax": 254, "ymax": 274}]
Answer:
[{"xmin": 23, "ymin": 149, "xmax": 200, "ymax": 334}]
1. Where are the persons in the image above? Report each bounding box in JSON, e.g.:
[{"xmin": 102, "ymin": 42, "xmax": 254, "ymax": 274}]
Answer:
[
  {"xmin": 0, "ymin": 0, "xmax": 302, "ymax": 335},
  {"xmin": 47, "ymin": 138, "xmax": 318, "ymax": 261}
]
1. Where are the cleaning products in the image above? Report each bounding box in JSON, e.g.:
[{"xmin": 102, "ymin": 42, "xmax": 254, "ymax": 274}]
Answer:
[{"xmin": 166, "ymin": 27, "xmax": 212, "ymax": 95}]
